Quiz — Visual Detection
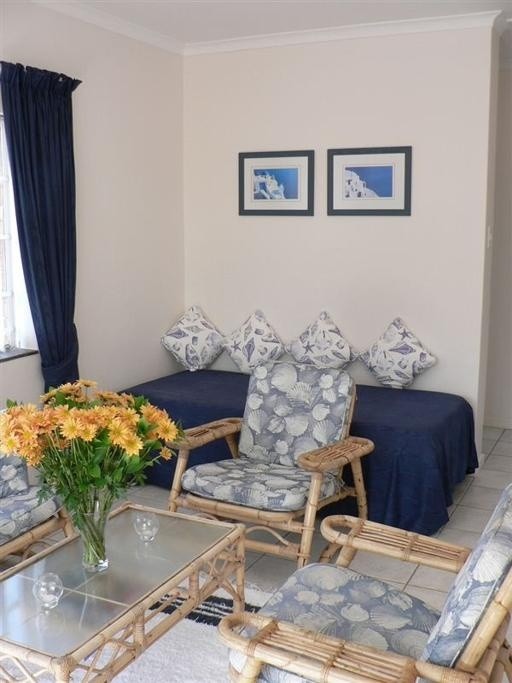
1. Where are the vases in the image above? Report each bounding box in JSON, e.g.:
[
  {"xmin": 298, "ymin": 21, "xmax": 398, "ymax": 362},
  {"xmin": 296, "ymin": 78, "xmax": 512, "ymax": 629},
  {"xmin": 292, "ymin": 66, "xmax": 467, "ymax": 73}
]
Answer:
[{"xmin": 71, "ymin": 508, "xmax": 111, "ymax": 573}]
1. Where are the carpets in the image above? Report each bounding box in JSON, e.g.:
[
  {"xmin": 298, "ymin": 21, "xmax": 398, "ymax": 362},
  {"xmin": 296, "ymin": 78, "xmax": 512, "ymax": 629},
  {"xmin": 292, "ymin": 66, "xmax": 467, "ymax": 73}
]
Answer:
[{"xmin": 10, "ymin": 543, "xmax": 274, "ymax": 682}]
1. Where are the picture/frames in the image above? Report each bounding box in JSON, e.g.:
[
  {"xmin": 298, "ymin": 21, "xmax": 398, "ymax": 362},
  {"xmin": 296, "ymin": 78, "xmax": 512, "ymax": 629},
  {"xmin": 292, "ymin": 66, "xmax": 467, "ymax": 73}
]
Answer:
[{"xmin": 238, "ymin": 144, "xmax": 413, "ymax": 217}]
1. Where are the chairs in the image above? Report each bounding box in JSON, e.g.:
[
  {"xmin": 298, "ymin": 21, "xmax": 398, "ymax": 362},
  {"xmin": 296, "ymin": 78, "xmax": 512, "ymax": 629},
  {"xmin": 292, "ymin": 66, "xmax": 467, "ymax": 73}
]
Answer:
[
  {"xmin": 169, "ymin": 363, "xmax": 379, "ymax": 565},
  {"xmin": 216, "ymin": 478, "xmax": 512, "ymax": 682}
]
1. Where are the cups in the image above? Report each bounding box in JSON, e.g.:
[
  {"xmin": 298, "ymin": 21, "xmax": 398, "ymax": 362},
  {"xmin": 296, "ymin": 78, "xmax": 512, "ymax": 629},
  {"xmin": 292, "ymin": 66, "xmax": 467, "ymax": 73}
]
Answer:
[
  {"xmin": 31, "ymin": 572, "xmax": 65, "ymax": 610},
  {"xmin": 133, "ymin": 511, "xmax": 161, "ymax": 542}
]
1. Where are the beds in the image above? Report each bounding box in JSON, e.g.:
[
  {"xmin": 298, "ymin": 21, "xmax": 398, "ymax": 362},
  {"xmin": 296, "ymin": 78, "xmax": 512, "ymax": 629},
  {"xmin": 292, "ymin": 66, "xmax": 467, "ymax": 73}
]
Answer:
[{"xmin": 117, "ymin": 363, "xmax": 474, "ymax": 530}]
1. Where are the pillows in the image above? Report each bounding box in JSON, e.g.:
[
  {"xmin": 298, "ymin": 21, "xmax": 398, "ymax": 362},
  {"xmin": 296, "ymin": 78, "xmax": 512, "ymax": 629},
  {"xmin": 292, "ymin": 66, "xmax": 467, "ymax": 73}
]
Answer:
[{"xmin": 160, "ymin": 302, "xmax": 437, "ymax": 392}]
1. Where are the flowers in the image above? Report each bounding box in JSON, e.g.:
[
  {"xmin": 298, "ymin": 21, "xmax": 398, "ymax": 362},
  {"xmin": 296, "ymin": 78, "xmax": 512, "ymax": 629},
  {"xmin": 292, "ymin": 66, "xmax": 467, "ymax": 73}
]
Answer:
[{"xmin": 0, "ymin": 377, "xmax": 184, "ymax": 563}]
